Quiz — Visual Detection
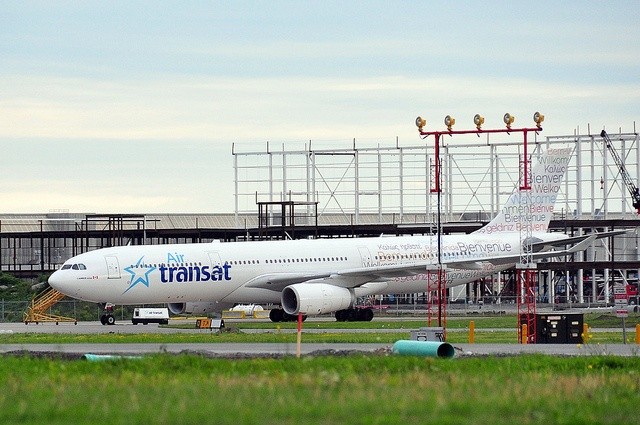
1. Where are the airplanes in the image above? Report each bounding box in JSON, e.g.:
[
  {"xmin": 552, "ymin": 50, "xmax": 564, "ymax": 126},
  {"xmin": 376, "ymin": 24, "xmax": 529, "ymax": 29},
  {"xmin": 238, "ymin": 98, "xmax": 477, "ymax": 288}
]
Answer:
[{"xmin": 48, "ymin": 149, "xmax": 627, "ymax": 327}]
